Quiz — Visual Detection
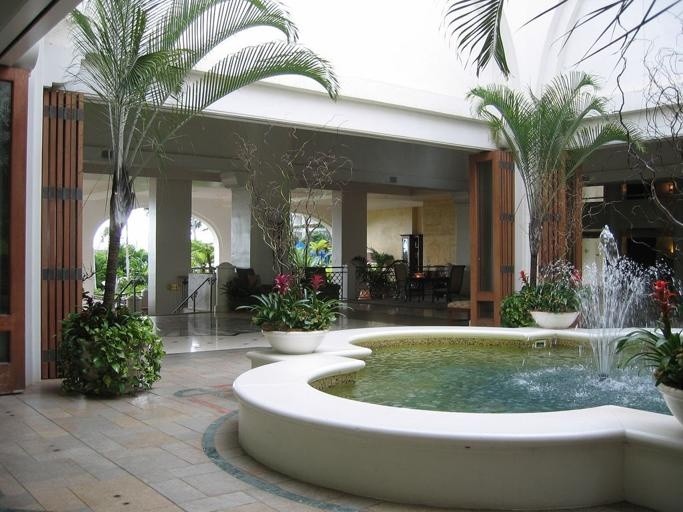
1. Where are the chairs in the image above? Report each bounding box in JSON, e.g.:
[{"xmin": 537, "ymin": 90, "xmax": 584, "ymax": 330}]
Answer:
[
  {"xmin": 300, "ymin": 266, "xmax": 340, "ymax": 306},
  {"xmin": 392, "ymin": 261, "xmax": 422, "ymax": 301},
  {"xmin": 235, "ymin": 267, "xmax": 276, "ymax": 307},
  {"xmin": 433, "ymin": 265, "xmax": 466, "ymax": 303}
]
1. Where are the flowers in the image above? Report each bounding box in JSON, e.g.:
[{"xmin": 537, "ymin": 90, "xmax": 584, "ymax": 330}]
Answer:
[
  {"xmin": 236, "ymin": 270, "xmax": 354, "ymax": 331},
  {"xmin": 608, "ymin": 278, "xmax": 683, "ymax": 391},
  {"xmin": 518, "ymin": 258, "xmax": 592, "ymax": 313}
]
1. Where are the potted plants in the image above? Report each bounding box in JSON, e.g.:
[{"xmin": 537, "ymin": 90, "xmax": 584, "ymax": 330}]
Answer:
[{"xmin": 53, "ymin": 298, "xmax": 165, "ymax": 398}]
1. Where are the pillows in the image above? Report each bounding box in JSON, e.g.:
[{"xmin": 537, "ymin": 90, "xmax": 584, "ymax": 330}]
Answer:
[{"xmin": 246, "ymin": 273, "xmax": 261, "ymax": 287}]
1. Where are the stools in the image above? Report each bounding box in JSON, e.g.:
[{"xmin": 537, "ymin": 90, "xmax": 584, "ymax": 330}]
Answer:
[{"xmin": 444, "ymin": 299, "xmax": 470, "ymax": 327}]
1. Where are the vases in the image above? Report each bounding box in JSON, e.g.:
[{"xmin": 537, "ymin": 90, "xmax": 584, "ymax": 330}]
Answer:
[
  {"xmin": 527, "ymin": 308, "xmax": 585, "ymax": 330},
  {"xmin": 651, "ymin": 368, "xmax": 683, "ymax": 427},
  {"xmin": 260, "ymin": 328, "xmax": 328, "ymax": 355}
]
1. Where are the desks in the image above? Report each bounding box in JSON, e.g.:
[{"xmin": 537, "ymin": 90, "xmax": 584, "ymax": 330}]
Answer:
[{"xmin": 407, "ymin": 276, "xmax": 448, "ymax": 304}]
